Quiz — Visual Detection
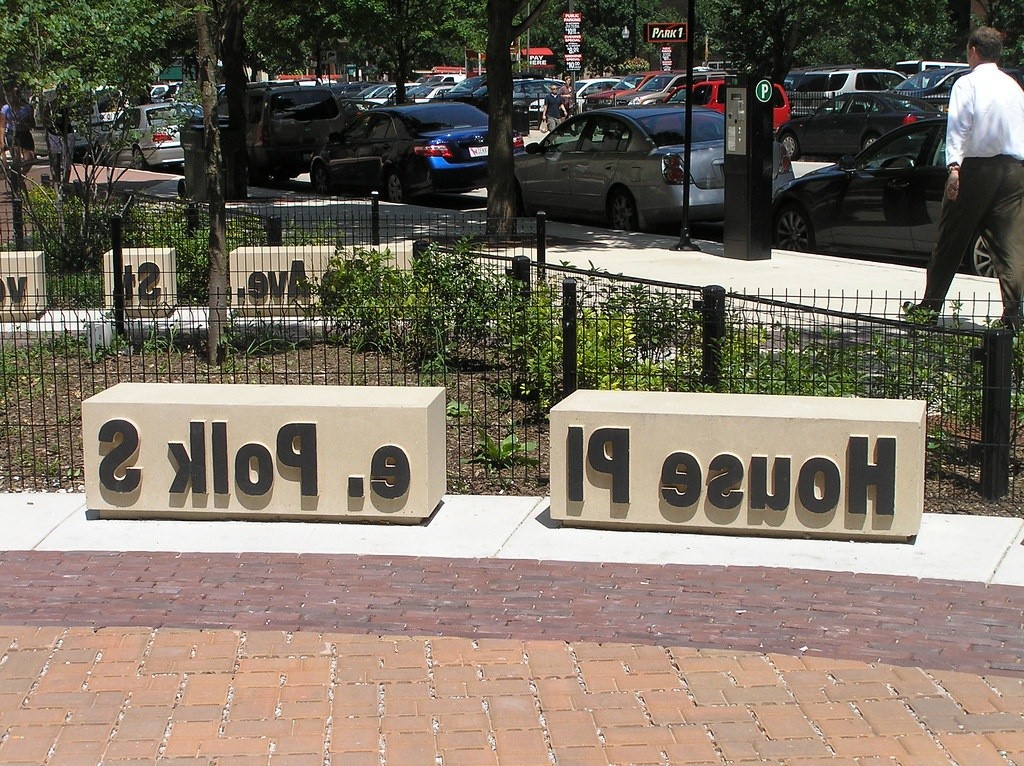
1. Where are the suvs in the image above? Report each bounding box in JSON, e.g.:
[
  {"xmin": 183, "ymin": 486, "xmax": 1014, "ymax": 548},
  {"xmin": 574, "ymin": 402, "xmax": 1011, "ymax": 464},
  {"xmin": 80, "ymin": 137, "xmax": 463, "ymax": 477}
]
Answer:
[{"xmin": 178, "ymin": 79, "xmax": 347, "ymax": 186}]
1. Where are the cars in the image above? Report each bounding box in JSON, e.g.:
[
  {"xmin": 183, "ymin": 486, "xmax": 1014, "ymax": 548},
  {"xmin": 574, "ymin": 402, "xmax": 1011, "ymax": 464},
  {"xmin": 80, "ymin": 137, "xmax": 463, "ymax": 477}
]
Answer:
[
  {"xmin": 511, "ymin": 106, "xmax": 795, "ymax": 232},
  {"xmin": 26, "ymin": 61, "xmax": 1024, "ymax": 168},
  {"xmin": 770, "ymin": 115, "xmax": 996, "ymax": 276},
  {"xmin": 308, "ymin": 102, "xmax": 528, "ymax": 203}
]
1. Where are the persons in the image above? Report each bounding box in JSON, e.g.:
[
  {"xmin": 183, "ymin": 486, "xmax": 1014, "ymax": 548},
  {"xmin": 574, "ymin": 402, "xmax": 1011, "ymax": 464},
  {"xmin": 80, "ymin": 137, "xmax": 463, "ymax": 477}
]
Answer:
[
  {"xmin": 0, "ymin": 83, "xmax": 76, "ymax": 193},
  {"xmin": 542, "ymin": 76, "xmax": 577, "ymax": 145},
  {"xmin": 903, "ymin": 25, "xmax": 1024, "ymax": 330}
]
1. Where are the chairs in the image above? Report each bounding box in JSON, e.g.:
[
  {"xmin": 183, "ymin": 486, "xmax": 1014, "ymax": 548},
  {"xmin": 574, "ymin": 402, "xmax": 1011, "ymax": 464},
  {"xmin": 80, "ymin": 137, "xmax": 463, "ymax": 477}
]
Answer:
[
  {"xmin": 603, "ymin": 132, "xmax": 622, "ymax": 143},
  {"xmin": 851, "ymin": 105, "xmax": 865, "ymax": 112},
  {"xmin": 878, "ymin": 103, "xmax": 896, "ymax": 113}
]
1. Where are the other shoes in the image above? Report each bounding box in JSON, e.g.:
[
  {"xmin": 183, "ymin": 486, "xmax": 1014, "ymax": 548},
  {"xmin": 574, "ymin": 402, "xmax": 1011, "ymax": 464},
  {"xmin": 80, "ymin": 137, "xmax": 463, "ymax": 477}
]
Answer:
[
  {"xmin": 994, "ymin": 320, "xmax": 1005, "ymax": 329},
  {"xmin": 904, "ymin": 301, "xmax": 937, "ymax": 325}
]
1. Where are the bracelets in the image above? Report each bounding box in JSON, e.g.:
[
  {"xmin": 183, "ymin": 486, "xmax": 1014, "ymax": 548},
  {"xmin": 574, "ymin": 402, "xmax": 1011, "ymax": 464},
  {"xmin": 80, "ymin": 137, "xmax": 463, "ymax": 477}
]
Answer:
[{"xmin": 948, "ymin": 165, "xmax": 961, "ymax": 173}]
